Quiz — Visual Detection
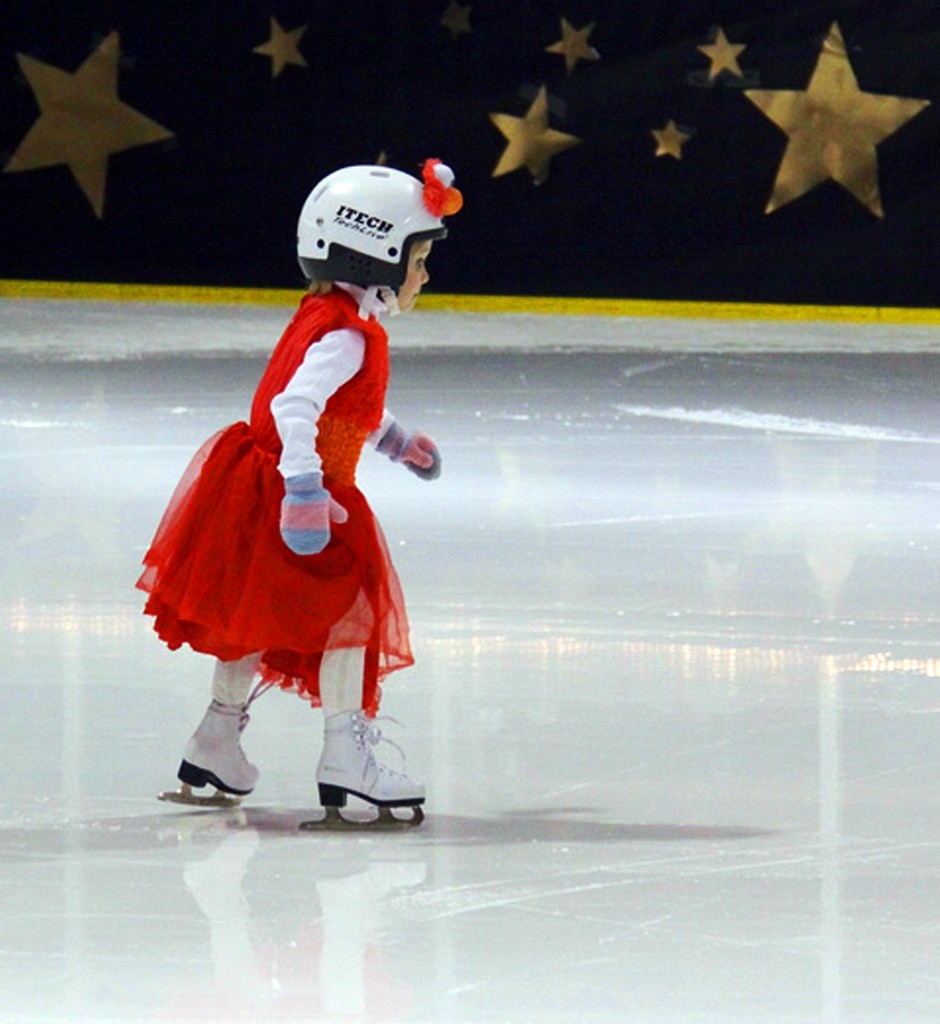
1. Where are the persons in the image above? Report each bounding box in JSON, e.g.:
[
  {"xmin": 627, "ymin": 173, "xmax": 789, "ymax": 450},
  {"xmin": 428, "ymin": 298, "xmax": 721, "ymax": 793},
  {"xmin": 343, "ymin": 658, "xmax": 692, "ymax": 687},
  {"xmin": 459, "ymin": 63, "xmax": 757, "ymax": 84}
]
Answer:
[{"xmin": 135, "ymin": 160, "xmax": 462, "ymax": 830}]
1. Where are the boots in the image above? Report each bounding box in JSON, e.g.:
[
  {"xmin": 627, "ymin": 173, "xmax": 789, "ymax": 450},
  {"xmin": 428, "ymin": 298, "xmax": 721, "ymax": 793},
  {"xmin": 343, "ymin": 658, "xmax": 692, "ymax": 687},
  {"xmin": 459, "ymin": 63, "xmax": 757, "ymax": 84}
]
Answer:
[
  {"xmin": 156, "ymin": 681, "xmax": 275, "ymax": 808},
  {"xmin": 297, "ymin": 710, "xmax": 426, "ymax": 834}
]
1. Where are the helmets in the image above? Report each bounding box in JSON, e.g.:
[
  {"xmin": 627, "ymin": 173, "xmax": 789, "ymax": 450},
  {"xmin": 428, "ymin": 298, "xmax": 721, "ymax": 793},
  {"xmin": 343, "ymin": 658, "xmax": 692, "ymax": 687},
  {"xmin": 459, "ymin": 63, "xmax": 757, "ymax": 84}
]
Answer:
[{"xmin": 298, "ymin": 165, "xmax": 447, "ymax": 290}]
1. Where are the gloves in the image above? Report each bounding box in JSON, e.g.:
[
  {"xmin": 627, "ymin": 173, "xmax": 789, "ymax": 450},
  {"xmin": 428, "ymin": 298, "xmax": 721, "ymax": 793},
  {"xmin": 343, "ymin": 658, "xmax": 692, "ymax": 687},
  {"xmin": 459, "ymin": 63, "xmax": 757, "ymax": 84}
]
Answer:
[
  {"xmin": 279, "ymin": 471, "xmax": 347, "ymax": 554},
  {"xmin": 374, "ymin": 425, "xmax": 439, "ymax": 480}
]
